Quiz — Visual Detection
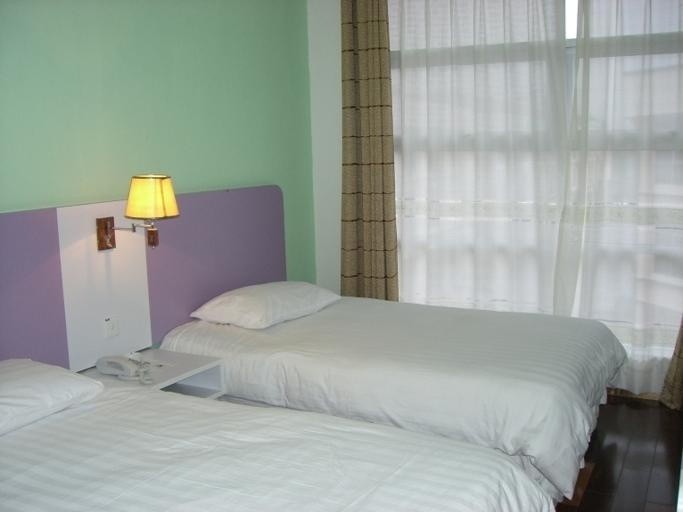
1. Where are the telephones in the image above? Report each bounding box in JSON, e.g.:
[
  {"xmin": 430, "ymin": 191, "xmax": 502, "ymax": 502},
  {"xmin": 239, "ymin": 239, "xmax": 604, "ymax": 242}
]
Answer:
[{"xmin": 96, "ymin": 354, "xmax": 154, "ymax": 384}]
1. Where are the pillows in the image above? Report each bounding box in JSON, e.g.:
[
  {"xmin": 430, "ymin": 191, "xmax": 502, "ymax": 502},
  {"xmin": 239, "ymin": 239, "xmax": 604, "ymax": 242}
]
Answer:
[
  {"xmin": 1, "ymin": 357, "xmax": 106, "ymax": 434},
  {"xmin": 189, "ymin": 281, "xmax": 343, "ymax": 330}
]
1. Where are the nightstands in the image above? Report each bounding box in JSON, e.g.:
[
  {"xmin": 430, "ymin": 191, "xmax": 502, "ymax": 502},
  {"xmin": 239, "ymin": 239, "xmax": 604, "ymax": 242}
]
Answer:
[{"xmin": 82, "ymin": 349, "xmax": 228, "ymax": 400}]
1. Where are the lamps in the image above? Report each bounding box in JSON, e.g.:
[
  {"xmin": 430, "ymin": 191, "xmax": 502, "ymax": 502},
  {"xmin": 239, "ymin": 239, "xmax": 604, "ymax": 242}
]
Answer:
[{"xmin": 96, "ymin": 174, "xmax": 181, "ymax": 252}]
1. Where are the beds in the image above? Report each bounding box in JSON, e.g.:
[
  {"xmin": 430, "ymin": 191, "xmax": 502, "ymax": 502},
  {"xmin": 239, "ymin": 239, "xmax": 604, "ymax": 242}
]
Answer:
[
  {"xmin": 146, "ymin": 293, "xmax": 626, "ymax": 501},
  {"xmin": 0, "ymin": 380, "xmax": 550, "ymax": 512}
]
